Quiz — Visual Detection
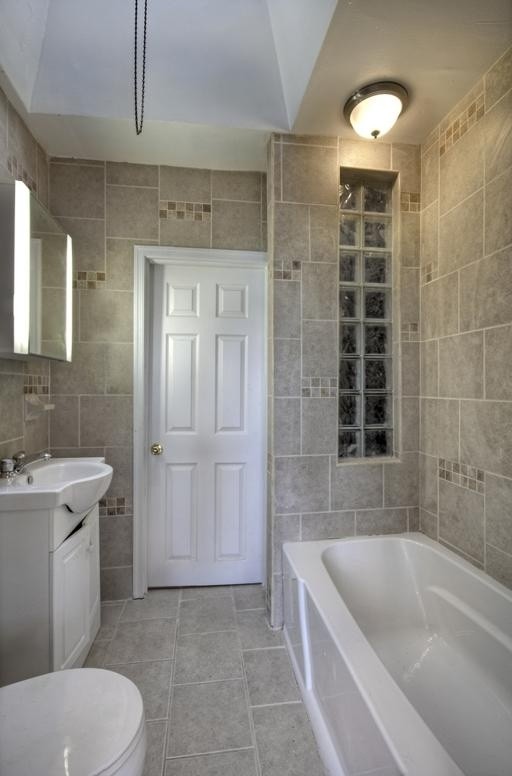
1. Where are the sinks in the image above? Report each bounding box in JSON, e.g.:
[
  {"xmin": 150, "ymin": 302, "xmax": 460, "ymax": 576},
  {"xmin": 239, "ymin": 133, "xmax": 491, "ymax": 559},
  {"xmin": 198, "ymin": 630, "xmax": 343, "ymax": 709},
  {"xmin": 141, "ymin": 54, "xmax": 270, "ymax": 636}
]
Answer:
[{"xmin": 17, "ymin": 460, "xmax": 113, "ymax": 513}]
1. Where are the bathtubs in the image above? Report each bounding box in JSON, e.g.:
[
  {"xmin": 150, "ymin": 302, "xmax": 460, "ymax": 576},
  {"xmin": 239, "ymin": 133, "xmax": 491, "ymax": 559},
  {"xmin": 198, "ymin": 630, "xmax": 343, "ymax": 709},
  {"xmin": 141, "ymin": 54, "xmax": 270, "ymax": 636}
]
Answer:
[{"xmin": 280, "ymin": 530, "xmax": 512, "ymax": 776}]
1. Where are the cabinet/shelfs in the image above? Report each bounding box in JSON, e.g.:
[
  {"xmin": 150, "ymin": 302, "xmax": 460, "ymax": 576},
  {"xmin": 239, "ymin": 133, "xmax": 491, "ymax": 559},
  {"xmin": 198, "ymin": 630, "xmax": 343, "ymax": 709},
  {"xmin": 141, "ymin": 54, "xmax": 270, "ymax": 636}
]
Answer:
[{"xmin": 0, "ymin": 500, "xmax": 102, "ymax": 688}]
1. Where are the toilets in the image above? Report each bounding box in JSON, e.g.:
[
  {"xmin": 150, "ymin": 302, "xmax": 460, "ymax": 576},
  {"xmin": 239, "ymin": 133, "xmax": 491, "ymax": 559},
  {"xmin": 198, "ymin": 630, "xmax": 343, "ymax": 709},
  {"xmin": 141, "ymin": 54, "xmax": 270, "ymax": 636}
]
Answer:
[{"xmin": 0, "ymin": 667, "xmax": 147, "ymax": 776}]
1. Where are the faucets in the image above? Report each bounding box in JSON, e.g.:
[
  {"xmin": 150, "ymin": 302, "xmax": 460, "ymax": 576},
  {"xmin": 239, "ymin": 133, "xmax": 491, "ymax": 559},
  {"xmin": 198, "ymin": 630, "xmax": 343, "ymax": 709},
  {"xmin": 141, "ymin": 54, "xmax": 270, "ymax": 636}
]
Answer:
[{"xmin": 12, "ymin": 449, "xmax": 52, "ymax": 474}]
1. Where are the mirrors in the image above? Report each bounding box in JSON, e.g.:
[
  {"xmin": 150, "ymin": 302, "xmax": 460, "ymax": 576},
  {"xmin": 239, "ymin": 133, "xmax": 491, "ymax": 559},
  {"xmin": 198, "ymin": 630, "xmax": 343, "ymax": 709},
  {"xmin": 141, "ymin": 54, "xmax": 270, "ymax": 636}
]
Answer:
[{"xmin": 13, "ymin": 178, "xmax": 74, "ymax": 365}]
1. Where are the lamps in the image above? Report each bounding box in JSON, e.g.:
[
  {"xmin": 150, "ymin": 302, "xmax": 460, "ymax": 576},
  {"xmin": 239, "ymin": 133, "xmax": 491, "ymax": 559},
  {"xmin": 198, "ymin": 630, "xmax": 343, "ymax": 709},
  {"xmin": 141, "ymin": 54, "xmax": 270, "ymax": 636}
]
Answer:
[{"xmin": 344, "ymin": 82, "xmax": 410, "ymax": 141}]
